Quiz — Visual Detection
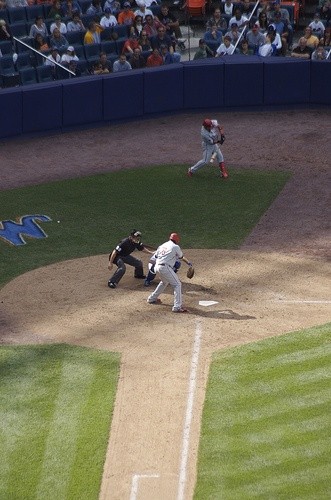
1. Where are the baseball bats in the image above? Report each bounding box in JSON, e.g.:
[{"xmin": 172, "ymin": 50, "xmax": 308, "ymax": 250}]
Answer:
[{"xmin": 209, "ymin": 143, "xmax": 222, "ymax": 163}]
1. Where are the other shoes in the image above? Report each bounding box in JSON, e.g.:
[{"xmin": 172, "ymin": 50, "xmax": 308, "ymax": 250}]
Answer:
[
  {"xmin": 149, "ymin": 299, "xmax": 161, "ymax": 304},
  {"xmin": 188, "ymin": 169, "xmax": 192, "ymax": 178},
  {"xmin": 220, "ymin": 171, "xmax": 228, "ymax": 177},
  {"xmin": 135, "ymin": 274, "xmax": 146, "ymax": 278},
  {"xmin": 173, "ymin": 308, "xmax": 187, "ymax": 313},
  {"xmin": 108, "ymin": 280, "xmax": 115, "ymax": 288},
  {"xmin": 145, "ymin": 280, "xmax": 150, "ymax": 287}
]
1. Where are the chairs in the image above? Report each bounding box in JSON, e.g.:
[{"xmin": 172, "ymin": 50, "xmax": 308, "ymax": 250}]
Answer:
[{"xmin": 0, "ymin": 0, "xmax": 331, "ymax": 89}]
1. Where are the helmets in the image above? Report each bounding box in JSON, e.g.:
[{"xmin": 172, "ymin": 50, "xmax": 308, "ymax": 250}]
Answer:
[{"xmin": 203, "ymin": 119, "xmax": 212, "ymax": 126}]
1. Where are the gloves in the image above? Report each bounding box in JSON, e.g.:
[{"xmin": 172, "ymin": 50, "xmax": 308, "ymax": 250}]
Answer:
[
  {"xmin": 217, "ymin": 140, "xmax": 224, "ymax": 145},
  {"xmin": 220, "ymin": 134, "xmax": 225, "ymax": 141}
]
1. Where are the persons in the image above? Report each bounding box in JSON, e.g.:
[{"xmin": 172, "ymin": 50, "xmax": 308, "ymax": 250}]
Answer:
[
  {"xmin": 145, "ymin": 254, "xmax": 181, "ymax": 286},
  {"xmin": 188, "ymin": 119, "xmax": 228, "ymax": 178},
  {"xmin": 108, "ymin": 230, "xmax": 154, "ymax": 289},
  {"xmin": 147, "ymin": 233, "xmax": 193, "ymax": 312},
  {"xmin": 0, "ymin": 0, "xmax": 186, "ymax": 75},
  {"xmin": 194, "ymin": 0, "xmax": 331, "ymax": 60}
]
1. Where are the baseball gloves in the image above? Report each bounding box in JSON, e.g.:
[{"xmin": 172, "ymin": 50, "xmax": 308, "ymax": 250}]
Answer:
[{"xmin": 187, "ymin": 266, "xmax": 195, "ymax": 279}]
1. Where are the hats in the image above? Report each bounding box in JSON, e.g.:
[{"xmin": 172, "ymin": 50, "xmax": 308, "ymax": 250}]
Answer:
[
  {"xmin": 67, "ymin": 46, "xmax": 74, "ymax": 52},
  {"xmin": 170, "ymin": 233, "xmax": 179, "ymax": 243},
  {"xmin": 131, "ymin": 229, "xmax": 141, "ymax": 236},
  {"xmin": 124, "ymin": 2, "xmax": 131, "ymax": 7},
  {"xmin": 231, "ymin": 23, "xmax": 238, "ymax": 27},
  {"xmin": 268, "ymin": 25, "xmax": 276, "ymax": 31},
  {"xmin": 105, "ymin": 7, "xmax": 111, "ymax": 14},
  {"xmin": 55, "ymin": 15, "xmax": 61, "ymax": 20}
]
